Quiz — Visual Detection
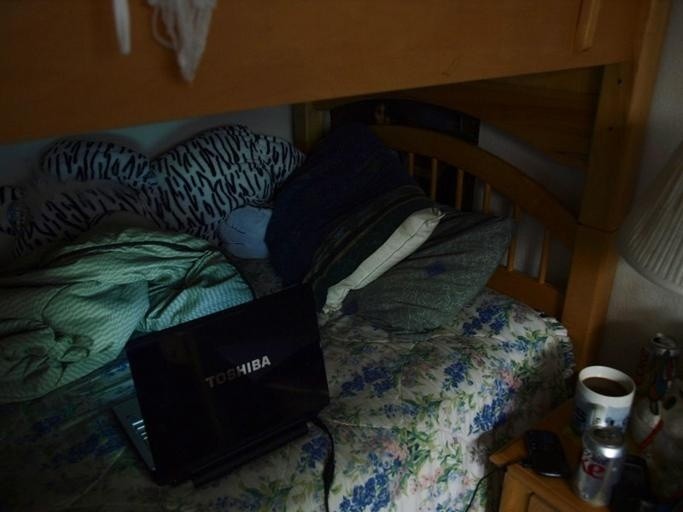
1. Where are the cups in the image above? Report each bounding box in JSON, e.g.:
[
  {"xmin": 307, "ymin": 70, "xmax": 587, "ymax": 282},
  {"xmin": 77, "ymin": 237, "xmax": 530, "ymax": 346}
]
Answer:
[{"xmin": 572, "ymin": 366, "xmax": 637, "ymax": 442}]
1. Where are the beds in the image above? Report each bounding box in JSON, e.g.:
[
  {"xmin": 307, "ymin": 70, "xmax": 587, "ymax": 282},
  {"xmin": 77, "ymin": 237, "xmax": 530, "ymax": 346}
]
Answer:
[{"xmin": 0, "ymin": 2, "xmax": 674, "ymax": 512}]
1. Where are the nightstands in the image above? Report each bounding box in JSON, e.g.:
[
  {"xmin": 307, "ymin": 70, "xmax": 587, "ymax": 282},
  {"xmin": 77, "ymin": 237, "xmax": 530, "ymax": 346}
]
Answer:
[{"xmin": 479, "ymin": 401, "xmax": 643, "ymax": 512}]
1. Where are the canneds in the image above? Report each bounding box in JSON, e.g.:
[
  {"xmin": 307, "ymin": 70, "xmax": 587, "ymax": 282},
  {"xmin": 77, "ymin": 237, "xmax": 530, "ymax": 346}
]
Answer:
[
  {"xmin": 636, "ymin": 331, "xmax": 681, "ymax": 399},
  {"xmin": 570, "ymin": 422, "xmax": 630, "ymax": 508}
]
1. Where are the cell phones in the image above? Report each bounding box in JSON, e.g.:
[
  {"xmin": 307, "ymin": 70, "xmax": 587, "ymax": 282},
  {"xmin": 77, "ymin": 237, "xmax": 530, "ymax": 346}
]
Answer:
[{"xmin": 522, "ymin": 428, "xmax": 570, "ymax": 478}]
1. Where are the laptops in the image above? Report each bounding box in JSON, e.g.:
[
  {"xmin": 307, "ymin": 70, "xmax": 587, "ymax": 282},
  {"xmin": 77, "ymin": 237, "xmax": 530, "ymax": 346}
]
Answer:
[{"xmin": 111, "ymin": 281, "xmax": 330, "ymax": 488}]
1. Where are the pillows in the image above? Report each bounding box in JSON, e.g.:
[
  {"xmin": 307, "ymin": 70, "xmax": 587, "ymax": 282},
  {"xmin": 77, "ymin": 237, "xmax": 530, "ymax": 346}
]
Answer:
[
  {"xmin": 337, "ymin": 204, "xmax": 516, "ymax": 338},
  {"xmin": 266, "ymin": 112, "xmax": 440, "ymax": 319}
]
